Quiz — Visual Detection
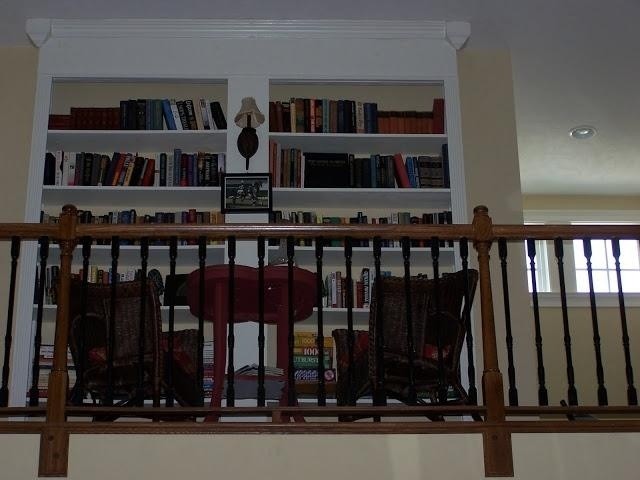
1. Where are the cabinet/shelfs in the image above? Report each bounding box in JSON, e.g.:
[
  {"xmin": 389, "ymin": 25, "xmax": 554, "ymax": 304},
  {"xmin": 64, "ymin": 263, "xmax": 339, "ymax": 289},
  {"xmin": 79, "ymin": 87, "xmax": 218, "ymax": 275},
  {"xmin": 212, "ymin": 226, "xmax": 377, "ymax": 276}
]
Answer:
[{"xmin": 21, "ymin": 63, "xmax": 459, "ymax": 415}]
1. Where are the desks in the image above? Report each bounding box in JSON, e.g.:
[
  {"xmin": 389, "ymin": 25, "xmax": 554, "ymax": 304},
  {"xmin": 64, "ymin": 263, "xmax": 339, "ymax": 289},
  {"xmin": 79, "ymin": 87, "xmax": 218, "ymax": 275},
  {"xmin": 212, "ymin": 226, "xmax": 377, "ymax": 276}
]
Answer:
[{"xmin": 181, "ymin": 263, "xmax": 327, "ymax": 427}]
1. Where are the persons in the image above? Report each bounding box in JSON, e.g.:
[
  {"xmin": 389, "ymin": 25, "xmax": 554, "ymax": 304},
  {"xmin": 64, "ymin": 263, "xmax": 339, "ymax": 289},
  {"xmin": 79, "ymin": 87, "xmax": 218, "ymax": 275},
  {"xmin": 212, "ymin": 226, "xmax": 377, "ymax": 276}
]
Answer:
[{"xmin": 232, "ymin": 183, "xmax": 259, "ymax": 206}]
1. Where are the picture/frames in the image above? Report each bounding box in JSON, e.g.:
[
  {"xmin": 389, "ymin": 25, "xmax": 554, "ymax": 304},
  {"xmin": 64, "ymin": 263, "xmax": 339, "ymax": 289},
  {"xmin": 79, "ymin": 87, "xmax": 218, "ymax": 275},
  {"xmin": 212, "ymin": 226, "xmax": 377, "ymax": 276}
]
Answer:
[{"xmin": 218, "ymin": 172, "xmax": 273, "ymax": 216}]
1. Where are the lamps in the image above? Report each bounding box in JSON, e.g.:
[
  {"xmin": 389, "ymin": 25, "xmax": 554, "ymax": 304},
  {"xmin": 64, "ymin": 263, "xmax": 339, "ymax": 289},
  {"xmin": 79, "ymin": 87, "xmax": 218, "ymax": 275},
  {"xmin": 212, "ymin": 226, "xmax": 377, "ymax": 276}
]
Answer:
[{"xmin": 233, "ymin": 95, "xmax": 269, "ymax": 172}]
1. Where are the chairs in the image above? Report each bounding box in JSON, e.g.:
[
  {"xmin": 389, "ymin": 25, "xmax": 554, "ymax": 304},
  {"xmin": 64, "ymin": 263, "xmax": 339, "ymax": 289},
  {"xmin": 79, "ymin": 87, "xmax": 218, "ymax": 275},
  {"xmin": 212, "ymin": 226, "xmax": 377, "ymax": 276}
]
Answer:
[
  {"xmin": 332, "ymin": 265, "xmax": 483, "ymax": 426},
  {"xmin": 47, "ymin": 262, "xmax": 205, "ymax": 425}
]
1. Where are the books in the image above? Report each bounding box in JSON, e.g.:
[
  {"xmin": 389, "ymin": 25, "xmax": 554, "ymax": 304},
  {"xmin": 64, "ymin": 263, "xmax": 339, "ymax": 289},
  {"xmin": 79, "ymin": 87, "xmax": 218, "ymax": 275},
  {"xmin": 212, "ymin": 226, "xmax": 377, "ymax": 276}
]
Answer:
[
  {"xmin": 44, "ymin": 139, "xmax": 451, "ymax": 189},
  {"xmin": 203, "ymin": 337, "xmax": 215, "ymax": 398},
  {"xmin": 322, "ymin": 268, "xmax": 390, "ymax": 309},
  {"xmin": 32, "ymin": 343, "xmax": 77, "ymax": 398},
  {"xmin": 410, "ymin": 273, "xmax": 428, "ymax": 280},
  {"xmin": 295, "ymin": 332, "xmax": 336, "ymax": 381},
  {"xmin": 273, "ymin": 211, "xmax": 454, "ymax": 248},
  {"xmin": 45, "ymin": 97, "xmax": 446, "ymax": 133},
  {"xmin": 39, "ymin": 210, "xmax": 225, "ymax": 245},
  {"xmin": 36, "ymin": 264, "xmax": 141, "ymax": 306},
  {"xmin": 236, "ymin": 362, "xmax": 285, "ymax": 377}
]
